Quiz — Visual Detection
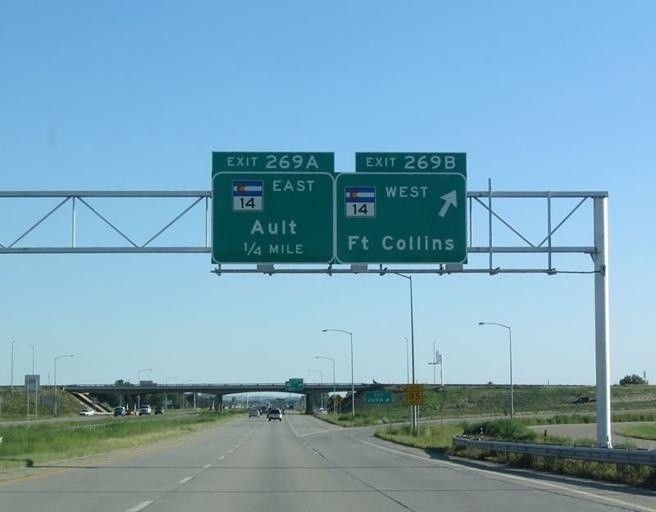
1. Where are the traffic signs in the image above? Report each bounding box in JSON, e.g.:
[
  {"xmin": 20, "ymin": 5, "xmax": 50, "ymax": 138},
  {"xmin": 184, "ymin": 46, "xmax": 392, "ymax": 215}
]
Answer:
[
  {"xmin": 407, "ymin": 391, "xmax": 423, "ymax": 405},
  {"xmin": 354, "ymin": 151, "xmax": 467, "ymax": 171},
  {"xmin": 211, "ymin": 150, "xmax": 335, "ymax": 172},
  {"xmin": 366, "ymin": 391, "xmax": 392, "ymax": 404},
  {"xmin": 334, "ymin": 171, "xmax": 468, "ymax": 260},
  {"xmin": 285, "ymin": 378, "xmax": 304, "ymax": 391},
  {"xmin": 213, "ymin": 173, "xmax": 333, "ymax": 263}
]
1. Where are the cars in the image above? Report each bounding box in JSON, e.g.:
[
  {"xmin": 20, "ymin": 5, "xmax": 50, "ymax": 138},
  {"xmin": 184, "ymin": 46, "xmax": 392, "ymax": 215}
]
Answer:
[
  {"xmin": 80, "ymin": 408, "xmax": 94, "ymax": 417},
  {"xmin": 114, "ymin": 405, "xmax": 165, "ymax": 418},
  {"xmin": 223, "ymin": 404, "xmax": 282, "ymax": 422}
]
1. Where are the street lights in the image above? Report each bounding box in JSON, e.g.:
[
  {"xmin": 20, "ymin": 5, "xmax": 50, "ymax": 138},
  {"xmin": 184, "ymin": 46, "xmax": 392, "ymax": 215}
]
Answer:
[
  {"xmin": 10, "ymin": 339, "xmax": 17, "ymax": 396},
  {"xmin": 307, "ymin": 369, "xmax": 323, "ymax": 383},
  {"xmin": 478, "ymin": 321, "xmax": 514, "ymax": 419},
  {"xmin": 30, "ymin": 343, "xmax": 34, "ymax": 382},
  {"xmin": 137, "ymin": 368, "xmax": 153, "ymax": 413},
  {"xmin": 315, "ymin": 356, "xmax": 336, "ymax": 415},
  {"xmin": 405, "ymin": 336, "xmax": 410, "ymax": 383},
  {"xmin": 322, "ymin": 329, "xmax": 355, "ymax": 417},
  {"xmin": 53, "ymin": 353, "xmax": 73, "ymax": 416}
]
